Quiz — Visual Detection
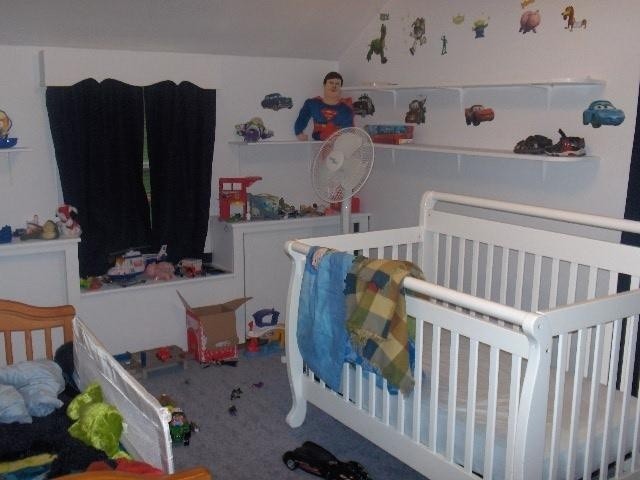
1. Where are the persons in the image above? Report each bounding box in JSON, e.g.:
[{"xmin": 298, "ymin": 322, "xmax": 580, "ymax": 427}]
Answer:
[{"xmin": 291, "ymin": 70, "xmax": 356, "ymax": 141}]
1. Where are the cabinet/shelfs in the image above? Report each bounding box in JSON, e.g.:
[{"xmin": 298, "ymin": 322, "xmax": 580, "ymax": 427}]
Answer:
[
  {"xmin": 211, "ymin": 213, "xmax": 374, "ymax": 342},
  {"xmin": 227, "ymin": 75, "xmax": 603, "ymax": 163},
  {"xmin": 0, "ymin": 236, "xmax": 80, "ymax": 363}
]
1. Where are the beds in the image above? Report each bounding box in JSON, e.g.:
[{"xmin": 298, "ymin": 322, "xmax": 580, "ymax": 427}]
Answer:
[
  {"xmin": 0, "ymin": 300, "xmax": 179, "ymax": 480},
  {"xmin": 285, "ymin": 191, "xmax": 640, "ymax": 480}
]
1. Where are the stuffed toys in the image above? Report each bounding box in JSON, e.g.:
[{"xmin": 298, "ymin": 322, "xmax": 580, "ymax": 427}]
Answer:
[
  {"xmin": 55, "ymin": 203, "xmax": 82, "ymax": 234},
  {"xmin": 227, "ymin": 404, "xmax": 238, "ymax": 417},
  {"xmin": 64, "ymin": 381, "xmax": 131, "ymax": 462},
  {"xmin": 19, "ymin": 219, "xmax": 60, "ymax": 241},
  {"xmin": 157, "ymin": 392, "xmax": 201, "ymax": 448},
  {"xmin": 233, "ymin": 117, "xmax": 275, "ymax": 143},
  {"xmin": 249, "ymin": 381, "xmax": 265, "ymax": 393},
  {"xmin": 230, "ymin": 387, "xmax": 244, "ymax": 400}
]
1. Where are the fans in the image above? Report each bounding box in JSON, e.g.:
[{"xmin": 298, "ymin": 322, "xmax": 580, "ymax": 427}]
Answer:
[{"xmin": 311, "ymin": 127, "xmax": 377, "ymax": 234}]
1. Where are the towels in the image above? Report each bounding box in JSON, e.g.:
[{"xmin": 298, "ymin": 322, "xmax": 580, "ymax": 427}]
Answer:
[
  {"xmin": 344, "ymin": 254, "xmax": 426, "ymax": 394},
  {"xmin": 295, "ymin": 245, "xmax": 418, "ymax": 393}
]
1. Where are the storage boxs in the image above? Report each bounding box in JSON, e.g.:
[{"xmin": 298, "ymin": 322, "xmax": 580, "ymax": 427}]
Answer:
[{"xmin": 175, "ymin": 288, "xmax": 254, "ymax": 363}]
